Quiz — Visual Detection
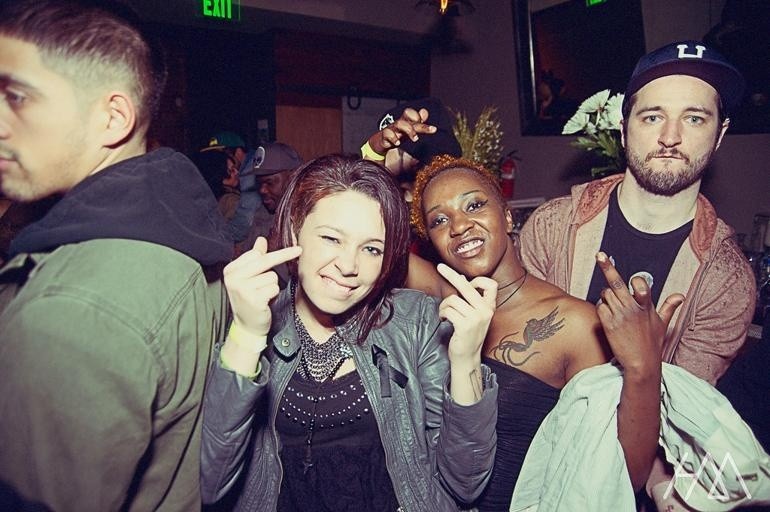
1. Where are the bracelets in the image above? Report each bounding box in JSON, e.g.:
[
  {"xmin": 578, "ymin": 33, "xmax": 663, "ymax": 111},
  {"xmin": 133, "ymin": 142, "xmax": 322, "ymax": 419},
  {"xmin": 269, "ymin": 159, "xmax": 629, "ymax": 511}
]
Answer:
[
  {"xmin": 218, "ymin": 345, "xmax": 262, "ymax": 381},
  {"xmin": 227, "ymin": 321, "xmax": 268, "ymax": 355},
  {"xmin": 360, "ymin": 141, "xmax": 384, "ymax": 162}
]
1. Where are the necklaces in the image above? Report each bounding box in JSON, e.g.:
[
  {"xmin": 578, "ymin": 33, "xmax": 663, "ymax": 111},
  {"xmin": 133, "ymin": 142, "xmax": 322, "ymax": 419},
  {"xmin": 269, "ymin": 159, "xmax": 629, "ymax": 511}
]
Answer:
[
  {"xmin": 494, "ymin": 266, "xmax": 529, "ymax": 309},
  {"xmin": 286, "ymin": 276, "xmax": 350, "ymax": 476}
]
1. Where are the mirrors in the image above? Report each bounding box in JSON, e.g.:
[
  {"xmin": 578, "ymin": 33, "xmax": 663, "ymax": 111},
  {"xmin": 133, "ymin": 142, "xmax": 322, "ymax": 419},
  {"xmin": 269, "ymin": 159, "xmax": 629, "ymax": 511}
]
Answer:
[{"xmin": 509, "ymin": 0, "xmax": 770, "ymax": 138}]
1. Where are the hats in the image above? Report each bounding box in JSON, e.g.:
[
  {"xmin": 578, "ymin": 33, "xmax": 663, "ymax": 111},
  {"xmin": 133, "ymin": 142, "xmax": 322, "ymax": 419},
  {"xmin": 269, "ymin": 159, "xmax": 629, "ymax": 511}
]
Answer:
[
  {"xmin": 376, "ymin": 94, "xmax": 462, "ymax": 166},
  {"xmin": 622, "ymin": 38, "xmax": 745, "ymax": 115},
  {"xmin": 239, "ymin": 143, "xmax": 300, "ymax": 176},
  {"xmin": 200, "ymin": 130, "xmax": 246, "ymax": 153}
]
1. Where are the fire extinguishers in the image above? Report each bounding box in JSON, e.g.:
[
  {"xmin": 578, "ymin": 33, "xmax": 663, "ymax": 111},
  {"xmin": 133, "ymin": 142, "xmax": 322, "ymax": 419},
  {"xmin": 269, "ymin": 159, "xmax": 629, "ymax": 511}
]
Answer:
[{"xmin": 499, "ymin": 147, "xmax": 522, "ymax": 200}]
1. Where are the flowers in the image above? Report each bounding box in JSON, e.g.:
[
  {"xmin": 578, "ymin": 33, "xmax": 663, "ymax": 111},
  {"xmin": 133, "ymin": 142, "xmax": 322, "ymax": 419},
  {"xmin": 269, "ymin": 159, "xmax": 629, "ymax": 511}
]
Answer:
[{"xmin": 562, "ymin": 89, "xmax": 626, "ymax": 176}]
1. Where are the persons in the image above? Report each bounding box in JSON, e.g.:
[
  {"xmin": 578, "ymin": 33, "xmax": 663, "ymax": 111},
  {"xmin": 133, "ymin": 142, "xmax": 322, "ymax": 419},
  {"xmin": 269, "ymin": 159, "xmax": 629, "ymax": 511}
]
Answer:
[
  {"xmin": 199, "ymin": 153, "xmax": 498, "ymax": 512},
  {"xmin": 360, "ymin": 107, "xmax": 687, "ymax": 512},
  {"xmin": 198, "ymin": 142, "xmax": 303, "ymax": 278},
  {"xmin": 518, "ymin": 37, "xmax": 757, "ymax": 392},
  {"xmin": 0, "ymin": 2, "xmax": 231, "ymax": 512}
]
